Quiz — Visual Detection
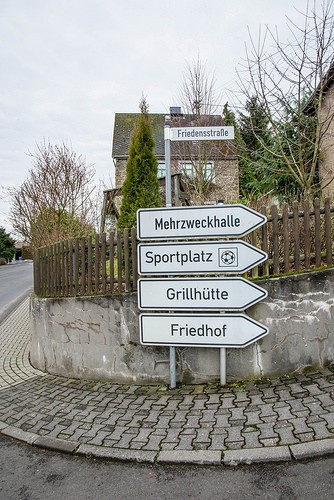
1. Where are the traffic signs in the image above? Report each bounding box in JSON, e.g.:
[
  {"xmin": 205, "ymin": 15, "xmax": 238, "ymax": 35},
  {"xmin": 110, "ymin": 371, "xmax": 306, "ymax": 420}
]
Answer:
[{"xmin": 134, "ymin": 204, "xmax": 270, "ymax": 349}]
